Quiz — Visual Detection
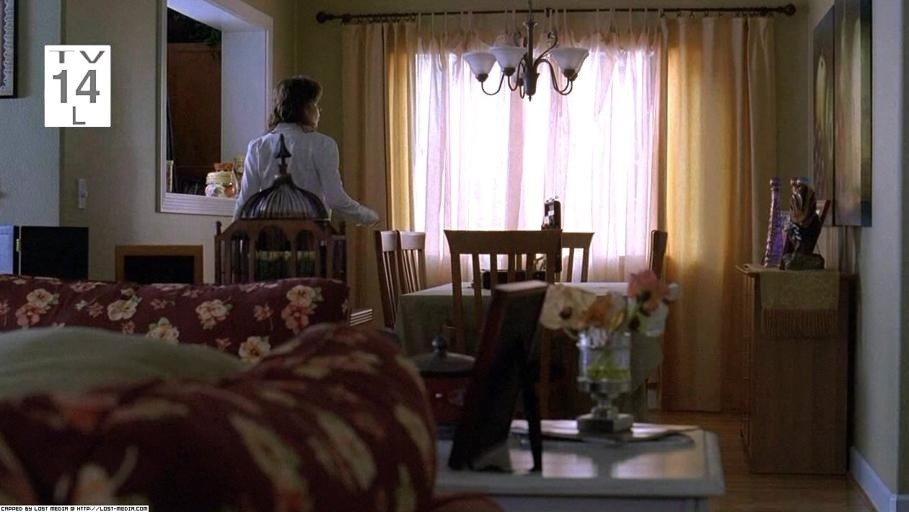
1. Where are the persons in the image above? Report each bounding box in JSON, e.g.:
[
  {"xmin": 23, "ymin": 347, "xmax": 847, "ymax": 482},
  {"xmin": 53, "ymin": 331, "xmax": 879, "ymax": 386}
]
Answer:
[{"xmin": 230, "ymin": 74, "xmax": 382, "ymax": 254}]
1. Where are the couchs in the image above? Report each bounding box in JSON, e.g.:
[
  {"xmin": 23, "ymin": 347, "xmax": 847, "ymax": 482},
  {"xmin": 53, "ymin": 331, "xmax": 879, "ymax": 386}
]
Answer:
[{"xmin": 0, "ymin": 258, "xmax": 435, "ymax": 512}]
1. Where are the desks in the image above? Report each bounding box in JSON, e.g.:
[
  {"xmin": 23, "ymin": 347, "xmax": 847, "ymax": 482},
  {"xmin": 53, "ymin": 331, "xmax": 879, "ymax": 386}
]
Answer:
[{"xmin": 430, "ymin": 409, "xmax": 728, "ymax": 512}]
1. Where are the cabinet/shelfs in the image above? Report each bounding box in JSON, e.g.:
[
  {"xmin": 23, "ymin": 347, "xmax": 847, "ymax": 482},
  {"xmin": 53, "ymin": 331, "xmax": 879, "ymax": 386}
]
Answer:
[{"xmin": 732, "ymin": 262, "xmax": 856, "ymax": 481}]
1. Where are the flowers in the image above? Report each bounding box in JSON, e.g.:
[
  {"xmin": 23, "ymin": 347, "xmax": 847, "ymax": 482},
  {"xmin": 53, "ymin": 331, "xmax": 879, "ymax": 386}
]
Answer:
[{"xmin": 538, "ymin": 268, "xmax": 680, "ymax": 396}]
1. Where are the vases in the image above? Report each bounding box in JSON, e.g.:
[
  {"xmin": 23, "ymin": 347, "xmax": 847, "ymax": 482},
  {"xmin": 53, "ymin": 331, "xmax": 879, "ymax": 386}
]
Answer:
[{"xmin": 577, "ymin": 333, "xmax": 640, "ymax": 431}]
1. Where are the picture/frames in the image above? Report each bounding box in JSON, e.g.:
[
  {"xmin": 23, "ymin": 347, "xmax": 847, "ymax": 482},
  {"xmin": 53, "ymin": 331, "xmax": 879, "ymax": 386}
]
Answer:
[{"xmin": 813, "ymin": 196, "xmax": 832, "ymax": 237}]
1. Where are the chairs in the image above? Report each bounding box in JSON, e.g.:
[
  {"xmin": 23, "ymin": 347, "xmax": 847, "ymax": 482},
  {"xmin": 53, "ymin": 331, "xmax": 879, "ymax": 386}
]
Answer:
[
  {"xmin": 443, "ymin": 228, "xmax": 564, "ymax": 320},
  {"xmin": 515, "ymin": 231, "xmax": 597, "ymax": 284},
  {"xmin": 372, "ymin": 228, "xmax": 404, "ymax": 331},
  {"xmin": 212, "ymin": 214, "xmax": 351, "ymax": 299},
  {"xmin": 646, "ymin": 229, "xmax": 669, "ymax": 281},
  {"xmin": 397, "ymin": 230, "xmax": 429, "ymax": 295}
]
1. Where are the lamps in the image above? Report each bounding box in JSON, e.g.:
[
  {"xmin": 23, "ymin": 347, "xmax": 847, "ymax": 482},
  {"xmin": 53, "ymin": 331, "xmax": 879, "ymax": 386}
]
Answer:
[{"xmin": 460, "ymin": 23, "xmax": 592, "ymax": 103}]
